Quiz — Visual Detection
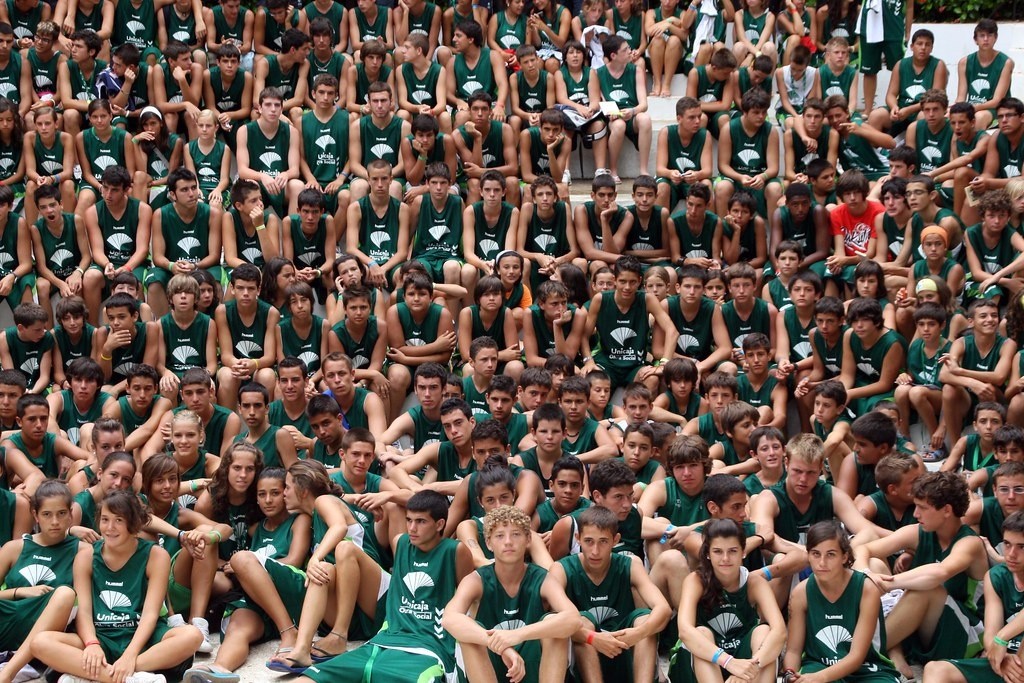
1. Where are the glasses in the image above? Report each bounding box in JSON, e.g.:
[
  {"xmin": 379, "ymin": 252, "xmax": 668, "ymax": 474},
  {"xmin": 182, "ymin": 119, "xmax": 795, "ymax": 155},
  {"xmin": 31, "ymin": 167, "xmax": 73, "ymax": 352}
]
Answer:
[
  {"xmin": 904, "ymin": 189, "xmax": 929, "ymax": 198},
  {"xmin": 34, "ymin": 33, "xmax": 54, "ymax": 43},
  {"xmin": 997, "ymin": 486, "xmax": 1024, "ymax": 494},
  {"xmin": 995, "ymin": 112, "xmax": 1019, "ymax": 120}
]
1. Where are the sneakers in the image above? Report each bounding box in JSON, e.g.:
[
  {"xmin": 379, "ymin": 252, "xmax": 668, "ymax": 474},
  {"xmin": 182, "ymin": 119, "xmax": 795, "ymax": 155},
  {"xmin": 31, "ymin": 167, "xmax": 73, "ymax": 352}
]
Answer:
[
  {"xmin": 562, "ymin": 170, "xmax": 573, "ymax": 184},
  {"xmin": 57, "ymin": 673, "xmax": 100, "ymax": 683},
  {"xmin": 612, "ymin": 176, "xmax": 622, "ymax": 184},
  {"xmin": 191, "ymin": 617, "xmax": 213, "ymax": 652},
  {"xmin": 167, "ymin": 613, "xmax": 190, "ymax": 627},
  {"xmin": 125, "ymin": 672, "xmax": 166, "ymax": 683},
  {"xmin": 595, "ymin": 168, "xmax": 612, "ymax": 176}
]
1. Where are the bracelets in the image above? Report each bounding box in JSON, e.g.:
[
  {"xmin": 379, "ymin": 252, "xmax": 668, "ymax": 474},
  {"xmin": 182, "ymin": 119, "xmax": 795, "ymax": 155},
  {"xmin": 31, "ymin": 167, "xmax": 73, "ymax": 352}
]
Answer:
[
  {"xmin": 131, "ymin": 137, "xmax": 139, "ymax": 144},
  {"xmin": 587, "ymin": 632, "xmax": 597, "ymax": 645},
  {"xmin": 0, "ymin": 181, "xmax": 4, "ymax": 186},
  {"xmin": 712, "ymin": 647, "xmax": 724, "ymax": 664},
  {"xmin": 85, "ymin": 640, "xmax": 100, "ymax": 648},
  {"xmin": 993, "ymin": 636, "xmax": 1009, "ymax": 647},
  {"xmin": 49, "ymin": 175, "xmax": 54, "ymax": 182},
  {"xmin": 721, "ymin": 654, "xmax": 732, "ymax": 667},
  {"xmin": 723, "ymin": 656, "xmax": 733, "ymax": 669},
  {"xmin": 210, "ymin": 530, "xmax": 221, "ymax": 543},
  {"xmin": 207, "ymin": 532, "xmax": 216, "ymax": 545},
  {"xmin": 14, "ymin": 587, "xmax": 21, "ymax": 599}
]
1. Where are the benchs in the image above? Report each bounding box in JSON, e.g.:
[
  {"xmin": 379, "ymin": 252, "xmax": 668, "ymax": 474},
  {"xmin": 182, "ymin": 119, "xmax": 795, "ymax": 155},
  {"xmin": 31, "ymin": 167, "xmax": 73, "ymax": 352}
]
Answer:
[{"xmin": 582, "ymin": 73, "xmax": 786, "ymax": 180}]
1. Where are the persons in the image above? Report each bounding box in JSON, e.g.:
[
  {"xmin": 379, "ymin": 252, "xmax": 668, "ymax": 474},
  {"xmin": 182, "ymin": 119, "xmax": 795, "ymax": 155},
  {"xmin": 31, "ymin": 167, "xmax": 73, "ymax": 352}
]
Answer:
[
  {"xmin": 923, "ymin": 508, "xmax": 1023, "ymax": 683},
  {"xmin": 0, "ymin": 0, "xmax": 1024, "ymax": 683},
  {"xmin": 667, "ymin": 518, "xmax": 787, "ymax": 683},
  {"xmin": 784, "ymin": 518, "xmax": 901, "ymax": 682},
  {"xmin": 549, "ymin": 506, "xmax": 673, "ymax": 683},
  {"xmin": 29, "ymin": 490, "xmax": 203, "ymax": 683},
  {"xmin": 288, "ymin": 489, "xmax": 474, "ymax": 683},
  {"xmin": 441, "ymin": 503, "xmax": 581, "ymax": 683},
  {"xmin": 0, "ymin": 480, "xmax": 93, "ymax": 682}
]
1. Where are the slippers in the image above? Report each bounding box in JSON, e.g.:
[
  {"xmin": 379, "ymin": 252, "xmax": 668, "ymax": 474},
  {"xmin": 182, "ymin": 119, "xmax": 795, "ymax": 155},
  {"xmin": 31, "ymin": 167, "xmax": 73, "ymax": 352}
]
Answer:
[
  {"xmin": 182, "ymin": 661, "xmax": 240, "ymax": 683},
  {"xmin": 266, "ymin": 647, "xmax": 293, "ymax": 668},
  {"xmin": 311, "ymin": 640, "xmax": 347, "ymax": 662},
  {"xmin": 269, "ymin": 657, "xmax": 311, "ymax": 673}
]
